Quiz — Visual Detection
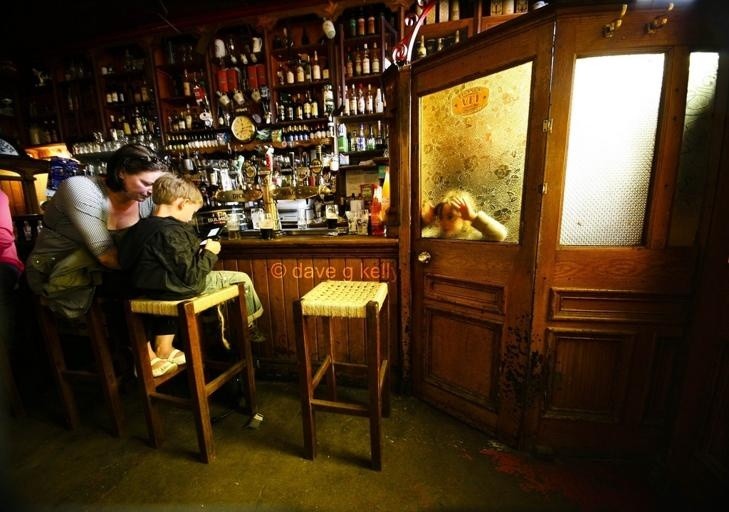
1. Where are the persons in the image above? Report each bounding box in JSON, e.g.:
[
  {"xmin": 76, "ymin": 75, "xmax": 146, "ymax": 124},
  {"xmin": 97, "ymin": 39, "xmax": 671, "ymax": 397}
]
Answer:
[
  {"xmin": 1, "ymin": 189, "xmax": 24, "ymax": 298},
  {"xmin": 119, "ymin": 170, "xmax": 267, "ymax": 345},
  {"xmin": 422, "ymin": 189, "xmax": 509, "ymax": 244},
  {"xmin": 24, "ymin": 141, "xmax": 187, "ymax": 378}
]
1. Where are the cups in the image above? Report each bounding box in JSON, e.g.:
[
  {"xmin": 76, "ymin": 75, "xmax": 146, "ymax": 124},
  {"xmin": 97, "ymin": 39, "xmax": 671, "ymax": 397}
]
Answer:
[
  {"xmin": 344, "ymin": 210, "xmax": 359, "ymax": 235},
  {"xmin": 258, "ymin": 213, "xmax": 274, "ymax": 241},
  {"xmin": 226, "ymin": 214, "xmax": 241, "ymax": 240},
  {"xmin": 232, "ymin": 89, "xmax": 246, "ymax": 105},
  {"xmin": 325, "ymin": 204, "xmax": 339, "ymax": 236},
  {"xmin": 218, "ymin": 91, "xmax": 232, "ymax": 105},
  {"xmin": 353, "ymin": 208, "xmax": 370, "ymax": 237},
  {"xmin": 276, "ymin": 87, "xmax": 321, "ymax": 122},
  {"xmin": 251, "ymin": 87, "xmax": 262, "ymax": 103}
]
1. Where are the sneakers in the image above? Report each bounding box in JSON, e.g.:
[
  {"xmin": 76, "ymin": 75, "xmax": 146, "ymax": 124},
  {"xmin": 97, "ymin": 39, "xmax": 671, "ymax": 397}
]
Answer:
[
  {"xmin": 134, "ymin": 358, "xmax": 177, "ymax": 379},
  {"xmin": 249, "ymin": 320, "xmax": 265, "ymax": 342},
  {"xmin": 161, "ymin": 349, "xmax": 187, "ymax": 365}
]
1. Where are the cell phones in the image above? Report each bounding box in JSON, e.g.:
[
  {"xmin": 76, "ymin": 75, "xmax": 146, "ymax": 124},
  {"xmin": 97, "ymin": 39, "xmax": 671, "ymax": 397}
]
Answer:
[{"xmin": 200, "ymin": 226, "xmax": 225, "ymax": 249}]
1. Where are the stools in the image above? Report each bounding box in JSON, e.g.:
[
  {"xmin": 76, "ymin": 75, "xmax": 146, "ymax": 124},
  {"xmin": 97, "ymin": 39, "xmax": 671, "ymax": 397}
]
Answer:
[
  {"xmin": 291, "ymin": 280, "xmax": 392, "ymax": 471},
  {"xmin": 38, "ymin": 284, "xmax": 140, "ymax": 443},
  {"xmin": 124, "ymin": 281, "xmax": 260, "ymax": 467}
]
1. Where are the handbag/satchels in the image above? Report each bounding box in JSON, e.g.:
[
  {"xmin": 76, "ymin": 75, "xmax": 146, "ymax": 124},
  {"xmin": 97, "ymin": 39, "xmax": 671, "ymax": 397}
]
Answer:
[{"xmin": 25, "ymin": 226, "xmax": 104, "ymax": 318}]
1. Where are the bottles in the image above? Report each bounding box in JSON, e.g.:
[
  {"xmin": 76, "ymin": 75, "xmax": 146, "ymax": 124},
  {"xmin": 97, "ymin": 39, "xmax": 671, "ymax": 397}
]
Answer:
[
  {"xmin": 371, "ymin": 185, "xmax": 386, "ymax": 236},
  {"xmin": 227, "ymin": 36, "xmax": 258, "ymax": 65},
  {"xmin": 338, "ymin": 118, "xmax": 390, "ymax": 154},
  {"xmin": 281, "ymin": 122, "xmax": 331, "ymax": 142},
  {"xmin": 62, "ymin": 86, "xmax": 81, "ymax": 111},
  {"xmin": 345, "ymin": 40, "xmax": 383, "ymax": 76},
  {"xmin": 99, "ymin": 45, "xmax": 146, "ymax": 72},
  {"xmin": 108, "ymin": 105, "xmax": 159, "ymax": 139},
  {"xmin": 165, "ymin": 39, "xmax": 200, "ymax": 64},
  {"xmin": 29, "ymin": 97, "xmax": 55, "ymax": 117},
  {"xmin": 167, "ymin": 67, "xmax": 207, "ymax": 98},
  {"xmin": 337, "ymin": 81, "xmax": 387, "ymax": 117},
  {"xmin": 163, "ymin": 103, "xmax": 208, "ymax": 131},
  {"xmin": 273, "ymin": 49, "xmax": 330, "ymax": 85},
  {"xmin": 61, "ymin": 54, "xmax": 89, "ymax": 79},
  {"xmin": 344, "ymin": 15, "xmax": 378, "ymax": 36},
  {"xmin": 102, "ymin": 75, "xmax": 153, "ymax": 105},
  {"xmin": 162, "ymin": 131, "xmax": 220, "ymax": 151},
  {"xmin": 414, "ymin": 29, "xmax": 461, "ymax": 59},
  {"xmin": 42, "ymin": 119, "xmax": 60, "ymax": 143}
]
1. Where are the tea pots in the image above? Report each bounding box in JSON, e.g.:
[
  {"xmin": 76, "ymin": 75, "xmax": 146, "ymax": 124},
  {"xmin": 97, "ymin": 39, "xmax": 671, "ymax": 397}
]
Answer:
[{"xmin": 252, "ymin": 35, "xmax": 262, "ymax": 53}]
